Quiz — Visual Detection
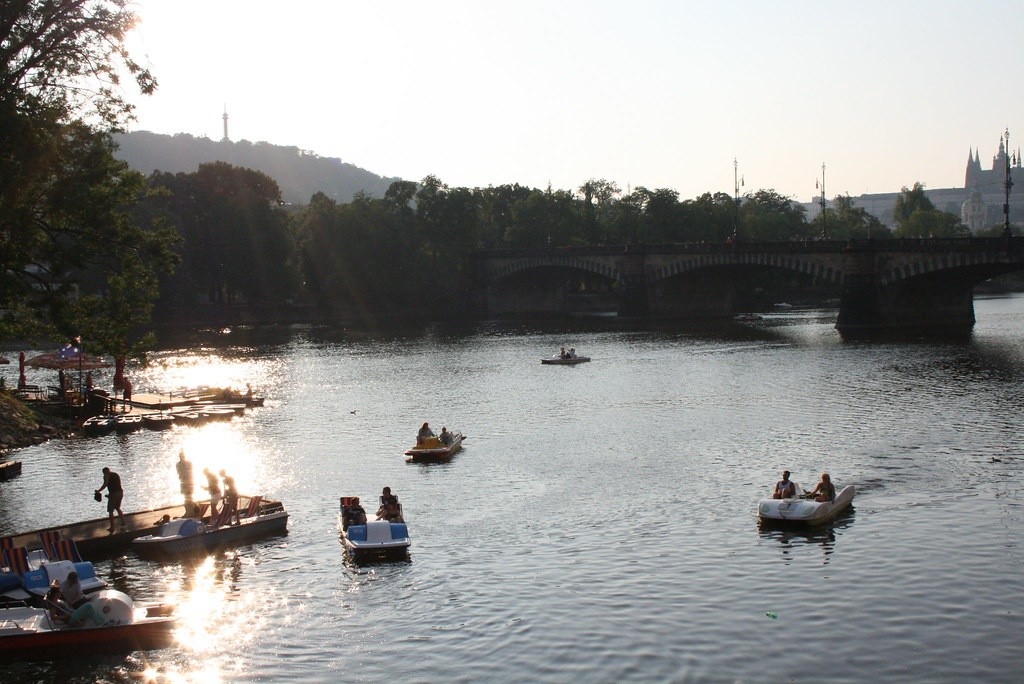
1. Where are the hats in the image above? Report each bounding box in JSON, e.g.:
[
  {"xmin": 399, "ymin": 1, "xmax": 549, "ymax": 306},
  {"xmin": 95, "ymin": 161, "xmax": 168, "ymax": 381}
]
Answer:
[{"xmin": 51, "ymin": 579, "xmax": 60, "ymax": 588}]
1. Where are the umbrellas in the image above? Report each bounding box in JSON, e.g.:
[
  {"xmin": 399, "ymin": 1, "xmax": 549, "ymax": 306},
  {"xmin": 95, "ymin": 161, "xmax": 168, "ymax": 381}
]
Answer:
[
  {"xmin": 19, "ymin": 350, "xmax": 26, "ymax": 386},
  {"xmin": 114, "ymin": 353, "xmax": 125, "ymax": 411},
  {"xmin": 23, "ymin": 343, "xmax": 111, "ymax": 377}
]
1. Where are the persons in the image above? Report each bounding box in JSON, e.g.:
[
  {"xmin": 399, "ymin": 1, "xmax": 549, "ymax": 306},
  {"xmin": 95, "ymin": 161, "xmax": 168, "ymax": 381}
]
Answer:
[
  {"xmin": 561, "ymin": 348, "xmax": 576, "ymax": 358},
  {"xmin": 86, "ymin": 372, "xmax": 94, "ymax": 391},
  {"xmin": 806, "ymin": 474, "xmax": 836, "ymax": 502},
  {"xmin": 47, "ymin": 586, "xmax": 120, "ymax": 627},
  {"xmin": 94, "ymin": 467, "xmax": 126, "ymax": 532},
  {"xmin": 61, "ymin": 572, "xmax": 100, "ymax": 611},
  {"xmin": 219, "ymin": 469, "xmax": 241, "ymax": 526},
  {"xmin": 122, "ymin": 377, "xmax": 133, "ymax": 412},
  {"xmin": 417, "ymin": 422, "xmax": 436, "ymax": 448},
  {"xmin": 341, "ymin": 497, "xmax": 367, "ymax": 532},
  {"xmin": 439, "ymin": 427, "xmax": 452, "ymax": 445},
  {"xmin": 375, "ymin": 486, "xmax": 402, "ymax": 523},
  {"xmin": 773, "ymin": 471, "xmax": 796, "ymax": 499},
  {"xmin": 199, "ymin": 468, "xmax": 222, "ymax": 523},
  {"xmin": 59, "ymin": 370, "xmax": 66, "ymax": 388}
]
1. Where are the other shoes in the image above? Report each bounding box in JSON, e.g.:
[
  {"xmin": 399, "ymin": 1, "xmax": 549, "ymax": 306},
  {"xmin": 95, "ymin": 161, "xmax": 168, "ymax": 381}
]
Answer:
[
  {"xmin": 107, "ymin": 528, "xmax": 114, "ymax": 533},
  {"xmin": 120, "ymin": 522, "xmax": 126, "ymax": 526}
]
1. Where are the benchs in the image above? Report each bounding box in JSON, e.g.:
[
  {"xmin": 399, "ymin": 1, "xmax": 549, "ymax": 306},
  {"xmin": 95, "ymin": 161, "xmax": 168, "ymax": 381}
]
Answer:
[
  {"xmin": 47, "ymin": 386, "xmax": 61, "ymax": 395},
  {"xmin": 17, "ymin": 385, "xmax": 43, "ymax": 400}
]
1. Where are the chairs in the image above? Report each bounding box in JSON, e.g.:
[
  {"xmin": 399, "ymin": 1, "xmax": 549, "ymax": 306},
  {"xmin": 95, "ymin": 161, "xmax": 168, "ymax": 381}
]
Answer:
[
  {"xmin": 380, "ymin": 495, "xmax": 399, "ymax": 504},
  {"xmin": 339, "ymin": 497, "xmax": 355, "ymax": 513},
  {"xmin": 51, "ymin": 539, "xmax": 83, "ymax": 562},
  {"xmin": 173, "ymin": 503, "xmax": 211, "ymax": 520},
  {"xmin": 48, "ymin": 577, "xmax": 61, "ymax": 591},
  {"xmin": 0, "ymin": 537, "xmax": 17, "ymax": 568},
  {"xmin": 35, "ymin": 531, "xmax": 63, "ymax": 562},
  {"xmin": 44, "ymin": 592, "xmax": 83, "ymax": 629},
  {"xmin": 4, "ymin": 546, "xmax": 32, "ymax": 572},
  {"xmin": 235, "ymin": 495, "xmax": 266, "ymax": 521},
  {"xmin": 212, "ymin": 498, "xmax": 234, "ymax": 528},
  {"xmin": 380, "ymin": 503, "xmax": 404, "ymax": 516}
]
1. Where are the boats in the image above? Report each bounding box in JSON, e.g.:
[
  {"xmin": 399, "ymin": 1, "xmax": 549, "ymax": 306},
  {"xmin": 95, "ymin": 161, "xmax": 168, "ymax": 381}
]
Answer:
[
  {"xmin": 756, "ymin": 482, "xmax": 855, "ymax": 528},
  {"xmin": 404, "ymin": 420, "xmax": 466, "ymax": 462},
  {"xmin": 733, "ymin": 312, "xmax": 762, "ymax": 320},
  {"xmin": 774, "ymin": 301, "xmax": 793, "ymax": 311},
  {"xmin": 130, "ymin": 495, "xmax": 291, "ymax": 556},
  {"xmin": 83, "ymin": 414, "xmax": 175, "ymax": 436},
  {"xmin": 337, "ymin": 496, "xmax": 410, "ymax": 562},
  {"xmin": 0, "ymin": 531, "xmax": 180, "ymax": 660},
  {"xmin": 541, "ymin": 355, "xmax": 591, "ymax": 365},
  {"xmin": 162, "ymin": 385, "xmax": 265, "ymax": 422}
]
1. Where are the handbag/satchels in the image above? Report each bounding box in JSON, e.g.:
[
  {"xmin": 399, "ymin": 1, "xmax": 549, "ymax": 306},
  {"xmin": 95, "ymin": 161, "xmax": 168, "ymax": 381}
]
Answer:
[{"xmin": 94, "ymin": 492, "xmax": 102, "ymax": 502}]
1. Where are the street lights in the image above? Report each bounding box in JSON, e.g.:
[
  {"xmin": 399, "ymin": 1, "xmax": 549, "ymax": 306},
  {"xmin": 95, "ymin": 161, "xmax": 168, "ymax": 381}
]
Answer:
[
  {"xmin": 815, "ymin": 158, "xmax": 826, "ymax": 240},
  {"xmin": 1002, "ymin": 128, "xmax": 1016, "ymax": 234},
  {"xmin": 733, "ymin": 158, "xmax": 744, "ymax": 240},
  {"xmin": 547, "ymin": 179, "xmax": 559, "ymax": 246}
]
